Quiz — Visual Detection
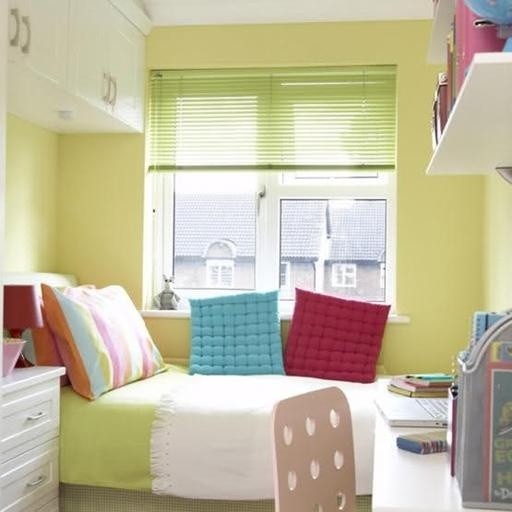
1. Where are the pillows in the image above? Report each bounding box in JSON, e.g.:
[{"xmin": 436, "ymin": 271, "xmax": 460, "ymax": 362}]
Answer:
[
  {"xmin": 283, "ymin": 287, "xmax": 391, "ymax": 383},
  {"xmin": 188, "ymin": 289, "xmax": 284, "ymax": 376},
  {"xmin": 22, "ymin": 327, "xmax": 70, "ymax": 387},
  {"xmin": 41, "ymin": 283, "xmax": 169, "ymax": 401}
]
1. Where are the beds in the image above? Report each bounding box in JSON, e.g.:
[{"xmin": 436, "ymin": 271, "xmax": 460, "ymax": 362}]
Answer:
[{"xmin": 1, "ymin": 271, "xmax": 451, "ymax": 501}]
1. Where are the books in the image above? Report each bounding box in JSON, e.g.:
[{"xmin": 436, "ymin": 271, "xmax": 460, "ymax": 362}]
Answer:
[
  {"xmin": 430, "ymin": 0, "xmax": 507, "ymax": 151},
  {"xmin": 378, "ymin": 369, "xmax": 455, "ymax": 430}
]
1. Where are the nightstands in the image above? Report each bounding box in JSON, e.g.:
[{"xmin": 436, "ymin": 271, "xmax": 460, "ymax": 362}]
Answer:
[{"xmin": 0, "ymin": 364, "xmax": 67, "ymax": 512}]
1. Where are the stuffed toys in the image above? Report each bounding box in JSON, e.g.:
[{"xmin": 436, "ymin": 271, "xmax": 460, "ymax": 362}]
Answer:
[{"xmin": 154, "ymin": 273, "xmax": 181, "ymax": 310}]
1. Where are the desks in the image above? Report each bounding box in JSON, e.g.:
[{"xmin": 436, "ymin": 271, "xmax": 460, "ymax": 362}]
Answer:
[{"xmin": 370, "ymin": 419, "xmax": 512, "ymax": 510}]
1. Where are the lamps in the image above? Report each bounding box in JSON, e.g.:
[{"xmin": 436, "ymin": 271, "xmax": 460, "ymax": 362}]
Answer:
[{"xmin": 3, "ymin": 284, "xmax": 44, "ymax": 368}]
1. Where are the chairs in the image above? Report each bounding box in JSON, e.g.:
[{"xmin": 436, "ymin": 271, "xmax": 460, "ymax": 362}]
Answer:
[{"xmin": 271, "ymin": 386, "xmax": 357, "ymax": 512}]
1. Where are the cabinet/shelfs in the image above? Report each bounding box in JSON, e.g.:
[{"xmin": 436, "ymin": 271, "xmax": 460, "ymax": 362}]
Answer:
[
  {"xmin": 426, "ymin": 0, "xmax": 512, "ymax": 176},
  {"xmin": 1, "ymin": 1, "xmax": 151, "ymax": 134}
]
